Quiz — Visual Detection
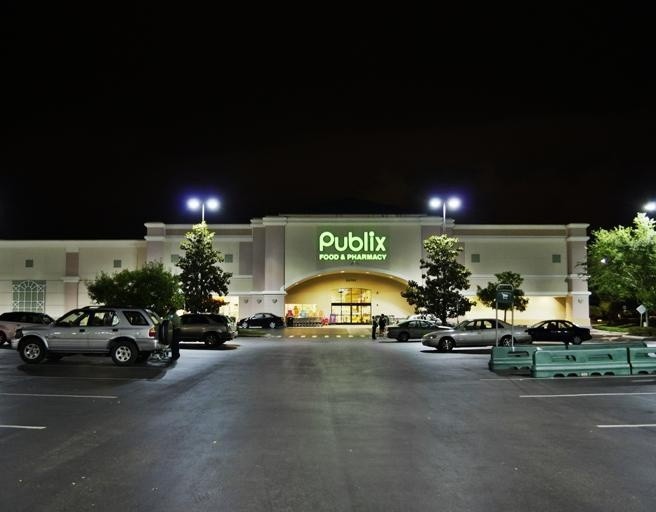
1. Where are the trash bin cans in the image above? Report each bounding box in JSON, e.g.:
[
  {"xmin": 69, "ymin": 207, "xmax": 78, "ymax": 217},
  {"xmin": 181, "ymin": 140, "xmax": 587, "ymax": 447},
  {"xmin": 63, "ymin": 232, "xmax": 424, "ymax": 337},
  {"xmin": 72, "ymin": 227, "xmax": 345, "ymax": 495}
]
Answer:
[{"xmin": 288, "ymin": 317, "xmax": 293, "ymax": 327}]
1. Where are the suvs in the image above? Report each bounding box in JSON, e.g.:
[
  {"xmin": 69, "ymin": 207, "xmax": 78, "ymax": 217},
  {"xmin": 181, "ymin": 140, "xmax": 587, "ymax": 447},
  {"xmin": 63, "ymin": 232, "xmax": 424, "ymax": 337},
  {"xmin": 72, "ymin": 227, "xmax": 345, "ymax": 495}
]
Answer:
[{"xmin": 0, "ymin": 305, "xmax": 241, "ymax": 368}]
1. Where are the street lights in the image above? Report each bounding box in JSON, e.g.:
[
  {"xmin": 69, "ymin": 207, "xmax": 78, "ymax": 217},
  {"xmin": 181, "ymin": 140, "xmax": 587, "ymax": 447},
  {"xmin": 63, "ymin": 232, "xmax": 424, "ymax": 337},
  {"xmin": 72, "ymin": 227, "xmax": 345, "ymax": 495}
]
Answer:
[
  {"xmin": 428, "ymin": 195, "xmax": 463, "ymax": 326},
  {"xmin": 185, "ymin": 196, "xmax": 223, "ymax": 222}
]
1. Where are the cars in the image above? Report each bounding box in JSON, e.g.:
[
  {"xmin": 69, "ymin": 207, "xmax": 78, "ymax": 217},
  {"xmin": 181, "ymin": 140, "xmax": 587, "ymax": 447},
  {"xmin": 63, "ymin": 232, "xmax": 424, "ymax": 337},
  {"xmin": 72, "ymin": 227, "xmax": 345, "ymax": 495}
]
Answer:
[
  {"xmin": 386, "ymin": 314, "xmax": 593, "ymax": 354},
  {"xmin": 240, "ymin": 312, "xmax": 285, "ymax": 330}
]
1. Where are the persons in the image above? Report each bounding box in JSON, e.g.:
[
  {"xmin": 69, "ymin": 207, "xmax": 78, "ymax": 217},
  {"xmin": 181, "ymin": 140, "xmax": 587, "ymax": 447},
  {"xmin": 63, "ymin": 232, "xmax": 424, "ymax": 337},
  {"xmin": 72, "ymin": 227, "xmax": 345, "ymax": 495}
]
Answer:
[
  {"xmin": 166, "ymin": 306, "xmax": 181, "ymax": 360},
  {"xmin": 371, "ymin": 315, "xmax": 379, "ymax": 340},
  {"xmin": 378, "ymin": 313, "xmax": 387, "ymax": 335}
]
